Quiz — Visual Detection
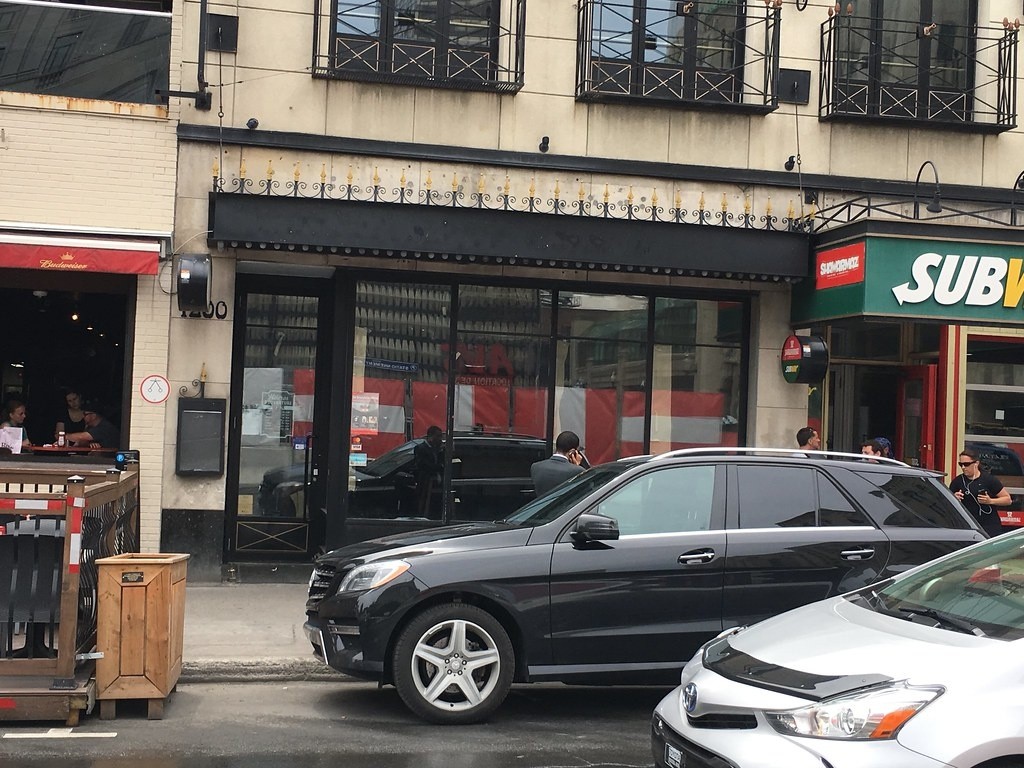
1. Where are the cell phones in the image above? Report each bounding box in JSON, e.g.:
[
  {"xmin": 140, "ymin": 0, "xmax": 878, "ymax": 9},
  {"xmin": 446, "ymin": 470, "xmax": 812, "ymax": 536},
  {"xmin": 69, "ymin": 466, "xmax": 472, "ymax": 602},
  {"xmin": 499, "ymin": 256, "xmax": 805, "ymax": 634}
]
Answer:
[
  {"xmin": 569, "ymin": 450, "xmax": 576, "ymax": 459},
  {"xmin": 978, "ymin": 489, "xmax": 986, "ymax": 496}
]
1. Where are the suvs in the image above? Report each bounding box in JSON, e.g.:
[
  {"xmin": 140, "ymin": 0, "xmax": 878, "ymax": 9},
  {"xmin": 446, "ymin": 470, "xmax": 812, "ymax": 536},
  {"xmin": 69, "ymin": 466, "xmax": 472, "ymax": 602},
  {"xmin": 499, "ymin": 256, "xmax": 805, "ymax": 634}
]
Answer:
[
  {"xmin": 258, "ymin": 431, "xmax": 591, "ymax": 521},
  {"xmin": 302, "ymin": 446, "xmax": 991, "ymax": 725}
]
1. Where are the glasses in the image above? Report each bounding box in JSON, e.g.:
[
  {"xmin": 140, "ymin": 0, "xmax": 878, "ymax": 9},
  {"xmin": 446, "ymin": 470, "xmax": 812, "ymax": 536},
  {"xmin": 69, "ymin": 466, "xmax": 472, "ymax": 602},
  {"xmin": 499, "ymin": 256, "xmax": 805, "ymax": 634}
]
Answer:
[
  {"xmin": 958, "ymin": 460, "xmax": 977, "ymax": 466},
  {"xmin": 83, "ymin": 412, "xmax": 96, "ymax": 417}
]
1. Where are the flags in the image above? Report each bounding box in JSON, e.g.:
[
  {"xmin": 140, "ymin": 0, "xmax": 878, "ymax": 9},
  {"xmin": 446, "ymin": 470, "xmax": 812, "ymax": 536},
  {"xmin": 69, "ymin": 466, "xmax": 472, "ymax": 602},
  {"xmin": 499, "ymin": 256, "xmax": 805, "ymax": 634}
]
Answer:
[
  {"xmin": 290, "ymin": 369, "xmax": 405, "ymax": 460},
  {"xmin": 553, "ymin": 386, "xmax": 723, "ymax": 466},
  {"xmin": 411, "ymin": 384, "xmax": 548, "ymax": 438}
]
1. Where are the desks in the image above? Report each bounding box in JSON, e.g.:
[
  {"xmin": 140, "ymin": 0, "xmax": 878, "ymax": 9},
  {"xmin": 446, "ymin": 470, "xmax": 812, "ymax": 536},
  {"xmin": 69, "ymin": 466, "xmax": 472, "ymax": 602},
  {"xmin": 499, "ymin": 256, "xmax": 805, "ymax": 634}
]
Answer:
[{"xmin": 22, "ymin": 446, "xmax": 119, "ymax": 456}]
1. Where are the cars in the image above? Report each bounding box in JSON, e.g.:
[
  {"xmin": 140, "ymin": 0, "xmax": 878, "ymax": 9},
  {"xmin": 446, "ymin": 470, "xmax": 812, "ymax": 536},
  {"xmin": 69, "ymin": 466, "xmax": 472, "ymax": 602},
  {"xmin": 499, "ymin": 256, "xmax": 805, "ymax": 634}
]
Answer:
[
  {"xmin": 965, "ymin": 441, "xmax": 1024, "ymax": 511},
  {"xmin": 652, "ymin": 526, "xmax": 1024, "ymax": 768}
]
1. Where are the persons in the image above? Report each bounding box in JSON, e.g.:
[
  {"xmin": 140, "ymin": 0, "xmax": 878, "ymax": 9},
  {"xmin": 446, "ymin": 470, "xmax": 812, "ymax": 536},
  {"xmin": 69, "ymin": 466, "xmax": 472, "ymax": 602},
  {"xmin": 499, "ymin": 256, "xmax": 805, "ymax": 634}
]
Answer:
[
  {"xmin": 55, "ymin": 402, "xmax": 118, "ymax": 448},
  {"xmin": 950, "ymin": 449, "xmax": 1012, "ymax": 537},
  {"xmin": 861, "ymin": 440, "xmax": 886, "ymax": 463},
  {"xmin": 412, "ymin": 426, "xmax": 444, "ymax": 518},
  {"xmin": 874, "ymin": 437, "xmax": 894, "ymax": 459},
  {"xmin": 0, "ymin": 400, "xmax": 31, "ymax": 453},
  {"xmin": 793, "ymin": 427, "xmax": 820, "ymax": 457},
  {"xmin": 55, "ymin": 390, "xmax": 86, "ymax": 447},
  {"xmin": 530, "ymin": 430, "xmax": 586, "ymax": 497}
]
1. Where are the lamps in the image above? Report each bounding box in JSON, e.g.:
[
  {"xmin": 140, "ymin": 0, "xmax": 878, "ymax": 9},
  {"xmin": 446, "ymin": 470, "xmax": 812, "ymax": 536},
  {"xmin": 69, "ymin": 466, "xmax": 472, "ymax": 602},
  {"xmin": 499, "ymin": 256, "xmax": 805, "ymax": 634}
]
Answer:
[
  {"xmin": 1011, "ymin": 170, "xmax": 1024, "ymax": 225},
  {"xmin": 913, "ymin": 160, "xmax": 942, "ymax": 220}
]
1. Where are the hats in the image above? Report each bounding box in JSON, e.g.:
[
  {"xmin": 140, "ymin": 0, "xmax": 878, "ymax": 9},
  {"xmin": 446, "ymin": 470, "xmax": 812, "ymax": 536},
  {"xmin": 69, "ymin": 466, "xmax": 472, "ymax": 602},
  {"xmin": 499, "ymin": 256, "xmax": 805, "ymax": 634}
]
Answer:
[
  {"xmin": 80, "ymin": 402, "xmax": 104, "ymax": 414},
  {"xmin": 64, "ymin": 379, "xmax": 82, "ymax": 399}
]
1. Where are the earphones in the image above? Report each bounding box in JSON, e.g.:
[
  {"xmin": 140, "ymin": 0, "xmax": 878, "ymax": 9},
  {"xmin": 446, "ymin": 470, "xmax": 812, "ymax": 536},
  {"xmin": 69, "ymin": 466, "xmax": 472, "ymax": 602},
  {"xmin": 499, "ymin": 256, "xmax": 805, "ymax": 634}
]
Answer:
[{"xmin": 976, "ymin": 464, "xmax": 977, "ymax": 465}]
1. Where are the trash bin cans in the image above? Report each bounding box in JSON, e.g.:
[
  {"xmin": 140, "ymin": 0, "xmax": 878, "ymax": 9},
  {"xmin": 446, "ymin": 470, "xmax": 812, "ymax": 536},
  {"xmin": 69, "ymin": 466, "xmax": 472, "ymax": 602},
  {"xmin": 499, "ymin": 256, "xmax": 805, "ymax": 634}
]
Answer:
[{"xmin": 94, "ymin": 552, "xmax": 190, "ymax": 700}]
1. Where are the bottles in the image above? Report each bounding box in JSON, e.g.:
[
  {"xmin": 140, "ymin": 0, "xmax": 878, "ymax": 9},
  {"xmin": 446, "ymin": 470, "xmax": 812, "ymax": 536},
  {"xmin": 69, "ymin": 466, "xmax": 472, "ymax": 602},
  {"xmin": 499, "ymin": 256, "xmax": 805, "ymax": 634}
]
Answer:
[
  {"xmin": 978, "ymin": 483, "xmax": 984, "ymax": 496},
  {"xmin": 58, "ymin": 432, "xmax": 66, "ymax": 448}
]
